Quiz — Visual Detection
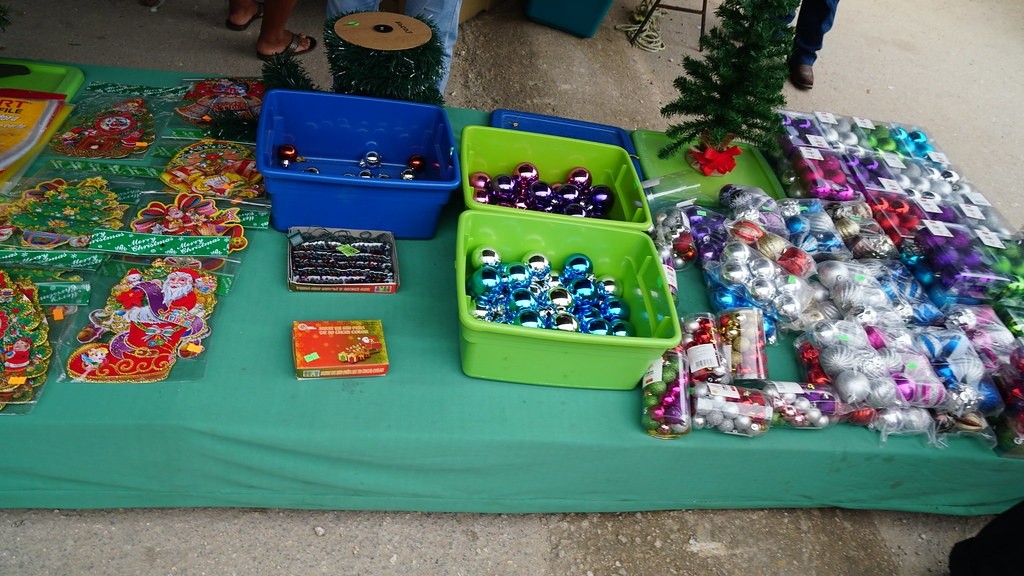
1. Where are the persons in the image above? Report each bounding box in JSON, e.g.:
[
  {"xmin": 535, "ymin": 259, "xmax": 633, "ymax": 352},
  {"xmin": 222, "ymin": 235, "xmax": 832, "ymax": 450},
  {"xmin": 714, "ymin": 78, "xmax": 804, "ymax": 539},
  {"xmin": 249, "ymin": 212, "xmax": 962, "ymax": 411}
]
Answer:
[
  {"xmin": 325, "ymin": 0, "xmax": 464, "ymax": 102},
  {"xmin": 750, "ymin": 0, "xmax": 839, "ymax": 93},
  {"xmin": 225, "ymin": 0, "xmax": 317, "ymax": 56}
]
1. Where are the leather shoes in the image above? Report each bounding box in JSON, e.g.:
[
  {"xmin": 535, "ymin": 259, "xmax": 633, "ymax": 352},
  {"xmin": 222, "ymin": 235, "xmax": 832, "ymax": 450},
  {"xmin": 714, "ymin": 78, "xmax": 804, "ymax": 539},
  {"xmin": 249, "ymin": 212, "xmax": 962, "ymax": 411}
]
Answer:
[{"xmin": 791, "ymin": 63, "xmax": 813, "ymax": 89}]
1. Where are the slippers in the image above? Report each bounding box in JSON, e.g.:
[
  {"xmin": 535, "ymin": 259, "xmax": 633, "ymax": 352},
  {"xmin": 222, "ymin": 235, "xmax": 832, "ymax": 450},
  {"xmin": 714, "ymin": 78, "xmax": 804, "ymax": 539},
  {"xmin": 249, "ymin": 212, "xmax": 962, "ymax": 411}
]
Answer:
[
  {"xmin": 225, "ymin": 2, "xmax": 264, "ymax": 31},
  {"xmin": 256, "ymin": 29, "xmax": 316, "ymax": 59}
]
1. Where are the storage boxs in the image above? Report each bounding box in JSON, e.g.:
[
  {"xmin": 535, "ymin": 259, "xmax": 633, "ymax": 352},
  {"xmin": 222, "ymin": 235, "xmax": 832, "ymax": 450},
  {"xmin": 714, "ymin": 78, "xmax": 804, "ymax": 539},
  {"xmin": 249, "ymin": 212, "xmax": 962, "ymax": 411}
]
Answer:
[
  {"xmin": 454, "ymin": 208, "xmax": 683, "ymax": 393},
  {"xmin": 255, "ymin": 88, "xmax": 463, "ymax": 243},
  {"xmin": 459, "ymin": 122, "xmax": 655, "ymax": 233}
]
